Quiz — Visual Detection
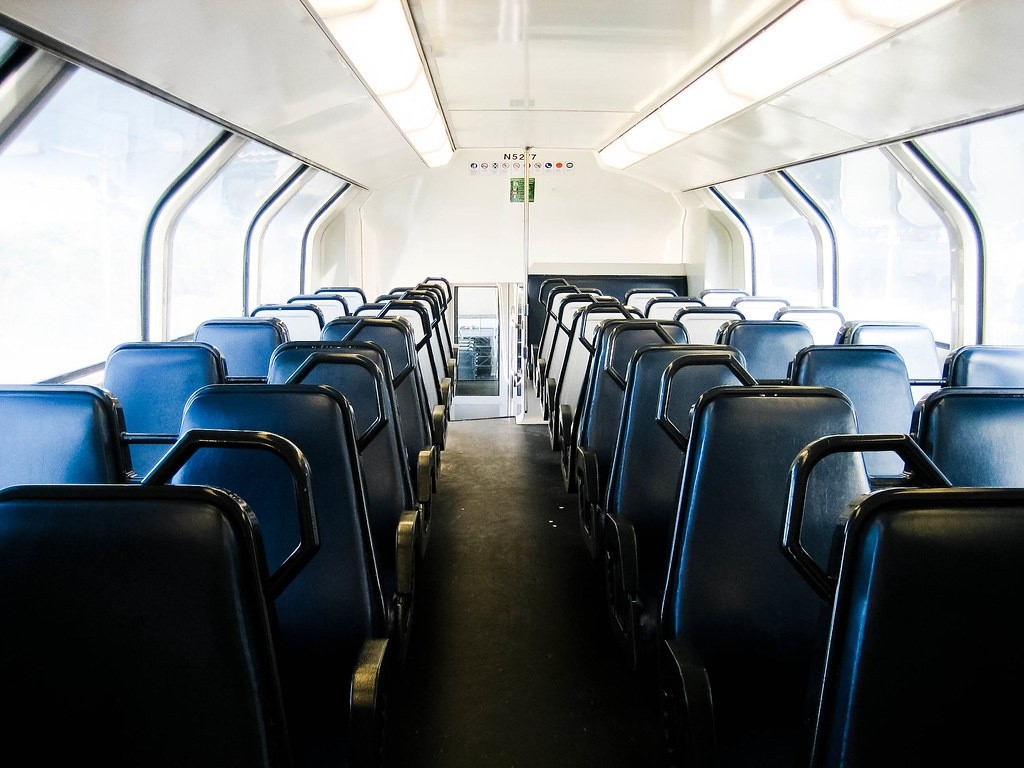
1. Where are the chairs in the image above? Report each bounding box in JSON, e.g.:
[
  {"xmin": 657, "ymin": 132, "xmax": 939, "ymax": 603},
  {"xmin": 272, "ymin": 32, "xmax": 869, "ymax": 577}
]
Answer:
[{"xmin": 0, "ymin": 276, "xmax": 1024, "ymax": 766}]
